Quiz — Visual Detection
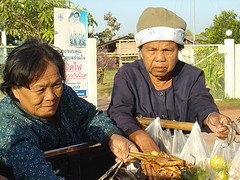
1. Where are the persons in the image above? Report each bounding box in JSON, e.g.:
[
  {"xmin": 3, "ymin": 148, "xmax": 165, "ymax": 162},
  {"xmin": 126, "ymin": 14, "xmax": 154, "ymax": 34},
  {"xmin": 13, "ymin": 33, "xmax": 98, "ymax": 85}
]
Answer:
[
  {"xmin": 0, "ymin": 38, "xmax": 138, "ymax": 180},
  {"xmin": 68, "ymin": 10, "xmax": 86, "ymax": 44},
  {"xmin": 106, "ymin": 7, "xmax": 234, "ymax": 163}
]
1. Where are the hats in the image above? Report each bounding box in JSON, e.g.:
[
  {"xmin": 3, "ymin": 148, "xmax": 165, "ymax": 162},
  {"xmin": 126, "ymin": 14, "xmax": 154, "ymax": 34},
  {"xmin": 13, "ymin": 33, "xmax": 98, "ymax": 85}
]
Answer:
[{"xmin": 135, "ymin": 7, "xmax": 186, "ymax": 48}]
